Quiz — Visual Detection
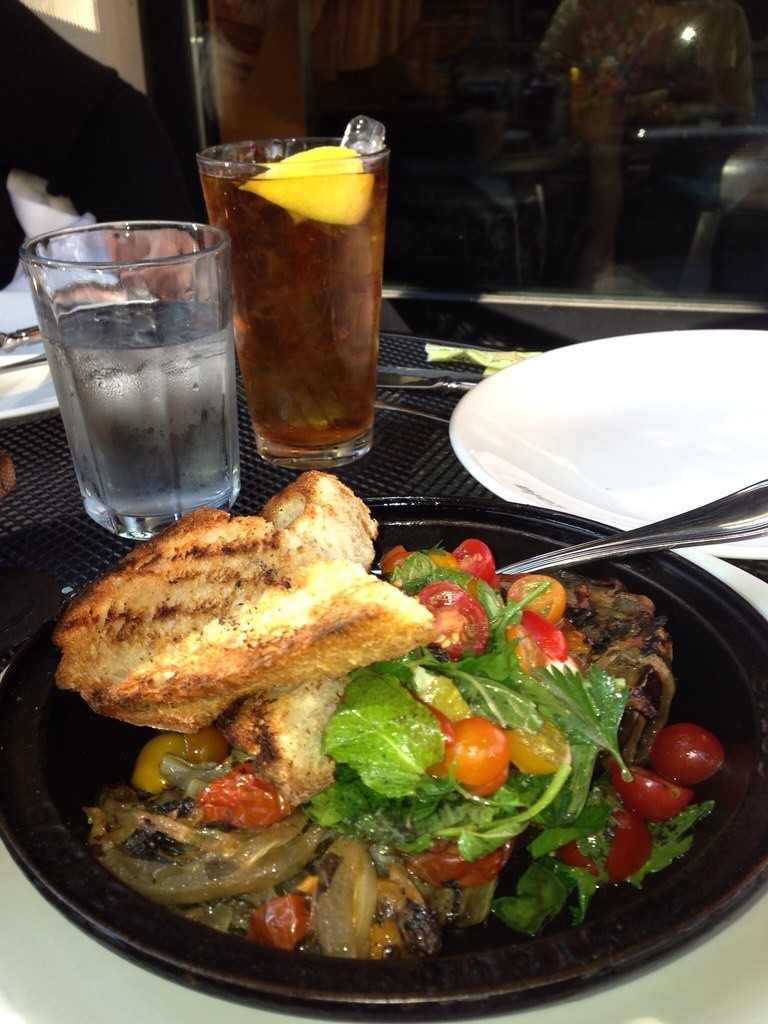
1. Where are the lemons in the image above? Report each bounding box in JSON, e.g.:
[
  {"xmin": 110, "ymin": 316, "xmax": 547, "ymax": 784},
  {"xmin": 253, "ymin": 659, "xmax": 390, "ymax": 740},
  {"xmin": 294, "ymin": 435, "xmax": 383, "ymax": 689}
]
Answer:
[{"xmin": 238, "ymin": 144, "xmax": 373, "ymax": 223}]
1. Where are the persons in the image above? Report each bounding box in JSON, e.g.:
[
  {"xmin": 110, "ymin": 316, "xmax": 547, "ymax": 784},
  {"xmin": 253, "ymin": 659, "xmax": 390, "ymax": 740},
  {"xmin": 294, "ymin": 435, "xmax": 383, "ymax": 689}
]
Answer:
[
  {"xmin": 537, "ymin": 0, "xmax": 756, "ymax": 126},
  {"xmin": 0, "ymin": 0, "xmax": 213, "ymax": 299}
]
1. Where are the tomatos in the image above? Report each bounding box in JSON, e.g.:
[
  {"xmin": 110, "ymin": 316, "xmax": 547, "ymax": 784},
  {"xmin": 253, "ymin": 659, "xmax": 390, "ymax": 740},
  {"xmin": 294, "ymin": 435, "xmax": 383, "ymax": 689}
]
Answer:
[
  {"xmin": 131, "ymin": 725, "xmax": 307, "ymax": 949},
  {"xmin": 377, "ymin": 539, "xmax": 724, "ymax": 885}
]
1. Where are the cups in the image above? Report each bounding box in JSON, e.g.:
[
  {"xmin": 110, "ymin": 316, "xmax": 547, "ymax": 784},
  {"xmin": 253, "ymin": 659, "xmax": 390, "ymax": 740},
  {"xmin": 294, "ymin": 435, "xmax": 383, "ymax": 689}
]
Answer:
[
  {"xmin": 19, "ymin": 220, "xmax": 241, "ymax": 542},
  {"xmin": 197, "ymin": 138, "xmax": 391, "ymax": 470}
]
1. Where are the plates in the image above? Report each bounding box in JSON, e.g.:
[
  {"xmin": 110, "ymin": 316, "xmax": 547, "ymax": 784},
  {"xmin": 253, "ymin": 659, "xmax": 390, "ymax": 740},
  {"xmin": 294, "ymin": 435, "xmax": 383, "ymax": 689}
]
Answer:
[
  {"xmin": 1, "ymin": 264, "xmax": 119, "ymax": 431},
  {"xmin": 450, "ymin": 328, "xmax": 768, "ymax": 560},
  {"xmin": 0, "ymin": 497, "xmax": 767, "ymax": 1023}
]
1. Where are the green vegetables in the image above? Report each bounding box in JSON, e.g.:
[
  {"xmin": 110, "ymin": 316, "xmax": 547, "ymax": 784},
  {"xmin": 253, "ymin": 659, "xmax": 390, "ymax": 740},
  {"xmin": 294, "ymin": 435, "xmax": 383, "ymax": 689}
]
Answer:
[{"xmin": 299, "ymin": 551, "xmax": 718, "ymax": 936}]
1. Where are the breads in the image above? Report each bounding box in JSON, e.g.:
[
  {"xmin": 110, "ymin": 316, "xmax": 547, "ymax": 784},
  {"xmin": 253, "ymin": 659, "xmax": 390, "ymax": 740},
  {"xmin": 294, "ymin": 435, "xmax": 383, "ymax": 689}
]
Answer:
[
  {"xmin": 53, "ymin": 506, "xmax": 436, "ymax": 733},
  {"xmin": 210, "ymin": 469, "xmax": 377, "ymax": 803}
]
